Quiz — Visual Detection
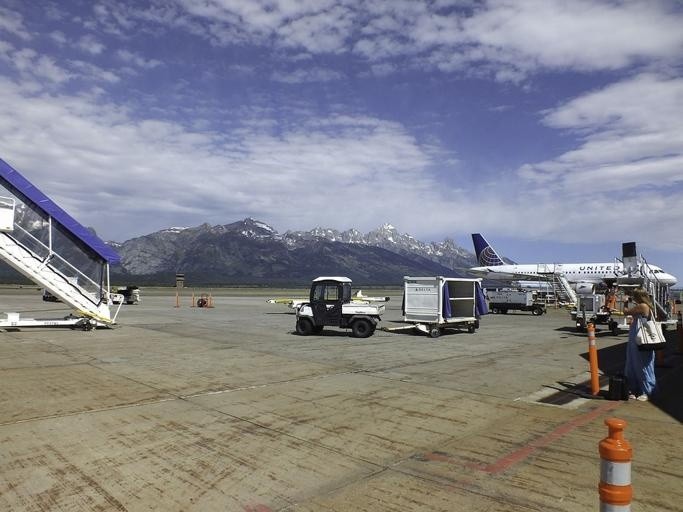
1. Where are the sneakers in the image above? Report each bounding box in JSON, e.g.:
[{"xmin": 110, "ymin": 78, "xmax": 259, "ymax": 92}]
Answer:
[{"xmin": 626, "ymin": 390, "xmax": 649, "ymax": 402}]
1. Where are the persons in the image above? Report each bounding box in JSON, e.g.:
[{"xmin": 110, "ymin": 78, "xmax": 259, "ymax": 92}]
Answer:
[{"xmin": 622, "ymin": 287, "xmax": 657, "ymax": 402}]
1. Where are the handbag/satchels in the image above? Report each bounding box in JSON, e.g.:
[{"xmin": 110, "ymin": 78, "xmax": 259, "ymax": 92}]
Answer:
[{"xmin": 634, "ymin": 320, "xmax": 667, "ymax": 346}]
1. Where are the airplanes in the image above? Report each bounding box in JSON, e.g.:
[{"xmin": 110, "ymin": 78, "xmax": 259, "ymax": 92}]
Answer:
[{"xmin": 467, "ymin": 232, "xmax": 678, "ymax": 309}]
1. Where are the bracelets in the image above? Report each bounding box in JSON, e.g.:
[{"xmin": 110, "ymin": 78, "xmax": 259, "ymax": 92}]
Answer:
[{"xmin": 623, "ymin": 301, "xmax": 628, "ymax": 308}]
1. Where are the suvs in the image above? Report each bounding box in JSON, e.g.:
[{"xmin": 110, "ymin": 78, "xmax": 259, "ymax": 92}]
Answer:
[{"xmin": 294, "ymin": 276, "xmax": 386, "ymax": 338}]
